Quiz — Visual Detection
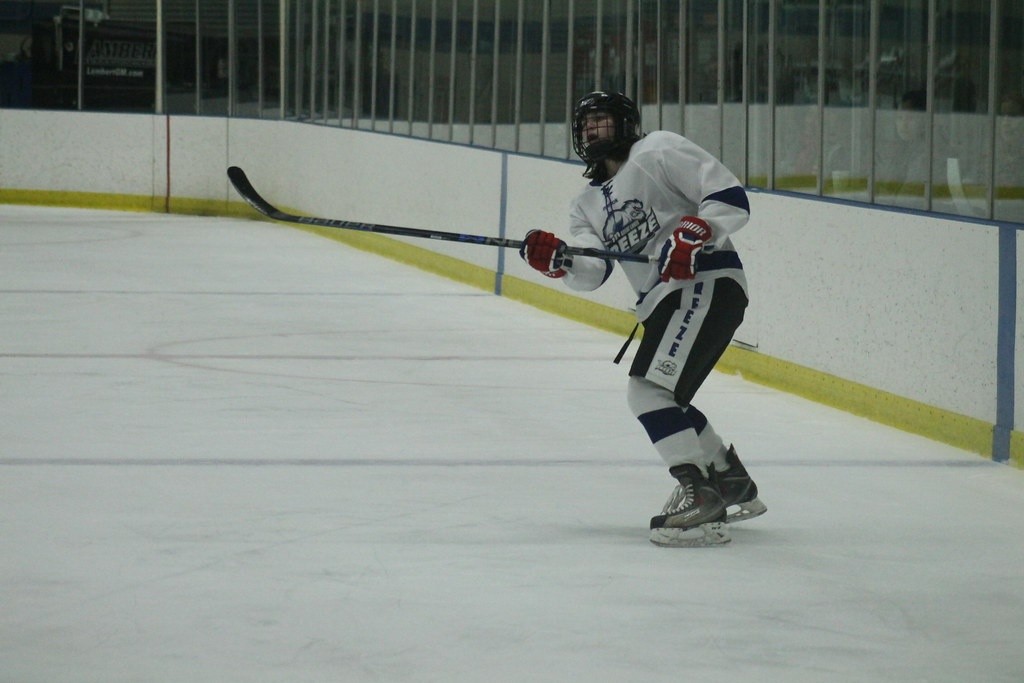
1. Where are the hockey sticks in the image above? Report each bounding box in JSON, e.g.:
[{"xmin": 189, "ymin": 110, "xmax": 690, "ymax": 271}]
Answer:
[{"xmin": 227, "ymin": 166, "xmax": 700, "ymax": 276}]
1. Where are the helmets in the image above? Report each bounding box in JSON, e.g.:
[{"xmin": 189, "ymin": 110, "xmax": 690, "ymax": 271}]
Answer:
[{"xmin": 573, "ymin": 90, "xmax": 639, "ymax": 162}]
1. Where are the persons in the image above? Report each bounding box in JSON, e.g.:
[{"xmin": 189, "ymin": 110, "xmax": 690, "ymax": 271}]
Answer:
[{"xmin": 519, "ymin": 89, "xmax": 769, "ymax": 545}]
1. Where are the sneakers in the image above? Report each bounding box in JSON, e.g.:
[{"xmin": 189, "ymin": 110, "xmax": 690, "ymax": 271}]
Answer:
[
  {"xmin": 705, "ymin": 443, "xmax": 768, "ymax": 523},
  {"xmin": 648, "ymin": 461, "xmax": 732, "ymax": 548}
]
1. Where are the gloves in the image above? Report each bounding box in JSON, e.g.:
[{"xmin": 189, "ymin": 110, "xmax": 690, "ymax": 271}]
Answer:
[
  {"xmin": 658, "ymin": 215, "xmax": 713, "ymax": 283},
  {"xmin": 519, "ymin": 229, "xmax": 574, "ymax": 278}
]
